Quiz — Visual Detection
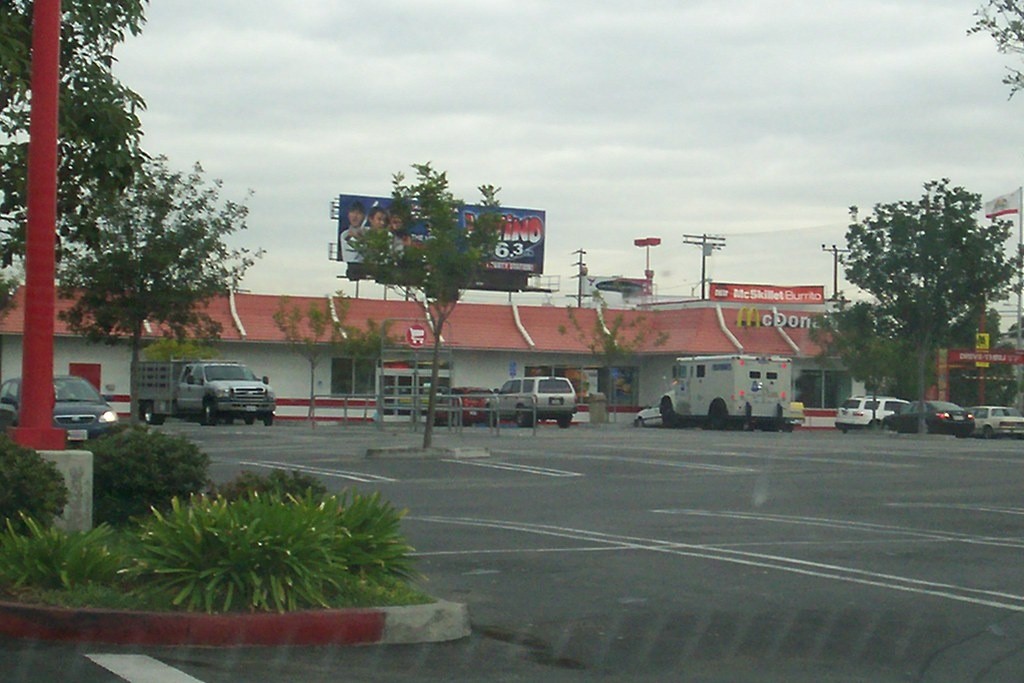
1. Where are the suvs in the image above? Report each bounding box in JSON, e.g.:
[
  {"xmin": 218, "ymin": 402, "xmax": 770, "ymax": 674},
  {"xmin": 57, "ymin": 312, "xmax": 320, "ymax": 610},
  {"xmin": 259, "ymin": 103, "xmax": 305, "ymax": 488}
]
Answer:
[
  {"xmin": 485, "ymin": 375, "xmax": 577, "ymax": 429},
  {"xmin": 834, "ymin": 394, "xmax": 910, "ymax": 433}
]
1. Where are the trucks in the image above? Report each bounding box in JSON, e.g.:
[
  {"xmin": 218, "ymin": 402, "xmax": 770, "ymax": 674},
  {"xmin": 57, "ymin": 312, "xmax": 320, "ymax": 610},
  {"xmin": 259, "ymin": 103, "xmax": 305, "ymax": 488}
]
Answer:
[
  {"xmin": 136, "ymin": 358, "xmax": 276, "ymax": 427},
  {"xmin": 659, "ymin": 354, "xmax": 793, "ymax": 432}
]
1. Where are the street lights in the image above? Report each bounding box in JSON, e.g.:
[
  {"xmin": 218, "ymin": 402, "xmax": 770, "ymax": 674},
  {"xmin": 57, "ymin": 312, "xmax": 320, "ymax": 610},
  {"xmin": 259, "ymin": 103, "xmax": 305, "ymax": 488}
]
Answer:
[{"xmin": 634, "ymin": 238, "xmax": 660, "ymax": 294}]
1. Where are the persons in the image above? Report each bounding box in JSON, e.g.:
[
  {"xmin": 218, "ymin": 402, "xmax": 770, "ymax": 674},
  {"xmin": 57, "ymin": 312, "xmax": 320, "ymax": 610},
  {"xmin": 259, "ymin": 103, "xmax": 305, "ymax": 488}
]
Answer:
[
  {"xmin": 341, "ymin": 201, "xmax": 372, "ymax": 261},
  {"xmin": 369, "ymin": 206, "xmax": 424, "ymax": 249}
]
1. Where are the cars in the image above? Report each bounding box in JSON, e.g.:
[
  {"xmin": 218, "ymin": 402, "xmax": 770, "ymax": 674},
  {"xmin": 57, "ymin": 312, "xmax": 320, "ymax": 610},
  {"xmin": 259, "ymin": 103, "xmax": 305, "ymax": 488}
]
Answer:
[
  {"xmin": 969, "ymin": 405, "xmax": 1024, "ymax": 440},
  {"xmin": 881, "ymin": 400, "xmax": 975, "ymax": 439},
  {"xmin": 0, "ymin": 375, "xmax": 118, "ymax": 445},
  {"xmin": 633, "ymin": 403, "xmax": 663, "ymax": 428},
  {"xmin": 434, "ymin": 386, "xmax": 494, "ymax": 426}
]
